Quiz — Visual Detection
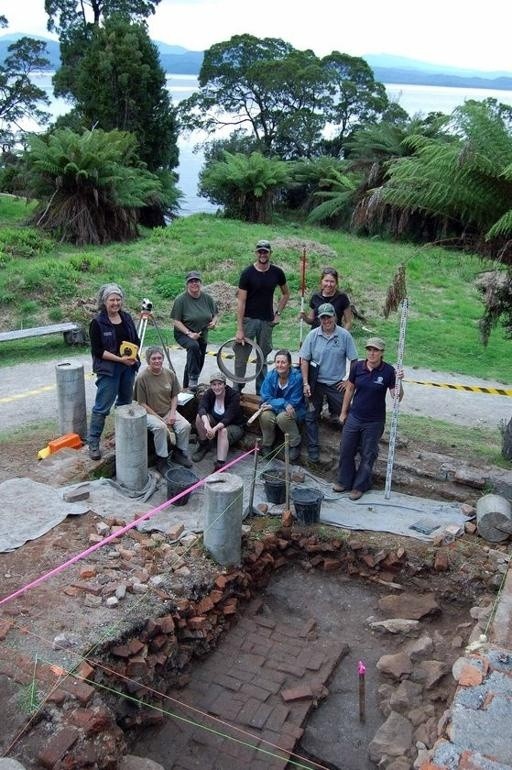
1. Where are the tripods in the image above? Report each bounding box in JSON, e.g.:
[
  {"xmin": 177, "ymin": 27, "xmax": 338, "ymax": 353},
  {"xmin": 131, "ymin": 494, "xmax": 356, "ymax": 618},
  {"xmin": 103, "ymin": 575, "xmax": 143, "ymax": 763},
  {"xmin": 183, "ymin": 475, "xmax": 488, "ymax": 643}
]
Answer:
[{"xmin": 137, "ymin": 316, "xmax": 174, "ymax": 372}]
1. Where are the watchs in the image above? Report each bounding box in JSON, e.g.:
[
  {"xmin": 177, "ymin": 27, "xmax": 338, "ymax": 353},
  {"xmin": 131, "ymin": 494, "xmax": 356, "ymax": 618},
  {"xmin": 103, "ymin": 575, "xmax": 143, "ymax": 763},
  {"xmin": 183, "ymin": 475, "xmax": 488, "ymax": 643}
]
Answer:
[{"xmin": 275, "ymin": 311, "xmax": 281, "ymax": 316}]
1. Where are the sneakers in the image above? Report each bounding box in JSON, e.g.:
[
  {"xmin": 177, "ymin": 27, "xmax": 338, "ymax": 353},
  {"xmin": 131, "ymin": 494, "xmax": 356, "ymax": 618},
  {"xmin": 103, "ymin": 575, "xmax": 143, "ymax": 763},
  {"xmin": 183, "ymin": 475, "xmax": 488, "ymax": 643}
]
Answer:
[
  {"xmin": 89, "ymin": 446, "xmax": 101, "ymax": 460},
  {"xmin": 188, "ymin": 375, "xmax": 198, "ymax": 391}
]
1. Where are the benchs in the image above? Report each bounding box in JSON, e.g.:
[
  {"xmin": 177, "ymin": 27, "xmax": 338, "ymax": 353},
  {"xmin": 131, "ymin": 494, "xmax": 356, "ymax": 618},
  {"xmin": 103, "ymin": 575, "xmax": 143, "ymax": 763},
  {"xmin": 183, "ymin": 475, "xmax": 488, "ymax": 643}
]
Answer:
[{"xmin": 1, "ymin": 321, "xmax": 84, "ymax": 362}]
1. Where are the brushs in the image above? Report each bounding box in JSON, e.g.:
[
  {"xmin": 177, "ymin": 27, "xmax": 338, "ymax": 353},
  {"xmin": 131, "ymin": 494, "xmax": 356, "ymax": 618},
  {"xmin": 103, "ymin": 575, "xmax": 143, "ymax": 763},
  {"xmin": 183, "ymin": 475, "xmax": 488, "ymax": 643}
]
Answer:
[
  {"xmin": 166, "ymin": 428, "xmax": 176, "ymax": 445},
  {"xmin": 246, "ymin": 402, "xmax": 267, "ymax": 427}
]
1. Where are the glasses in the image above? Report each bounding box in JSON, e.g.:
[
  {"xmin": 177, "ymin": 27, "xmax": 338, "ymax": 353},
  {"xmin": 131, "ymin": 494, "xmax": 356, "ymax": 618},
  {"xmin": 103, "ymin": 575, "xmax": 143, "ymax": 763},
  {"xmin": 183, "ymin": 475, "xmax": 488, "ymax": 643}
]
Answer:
[{"xmin": 321, "ymin": 268, "xmax": 338, "ymax": 277}]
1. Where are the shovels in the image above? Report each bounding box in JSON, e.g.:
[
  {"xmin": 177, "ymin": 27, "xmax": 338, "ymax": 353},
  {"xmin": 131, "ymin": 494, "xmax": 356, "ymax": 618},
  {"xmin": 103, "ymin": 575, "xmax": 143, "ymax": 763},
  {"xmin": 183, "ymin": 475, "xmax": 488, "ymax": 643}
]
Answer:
[{"xmin": 198, "ymin": 324, "xmax": 209, "ymax": 334}]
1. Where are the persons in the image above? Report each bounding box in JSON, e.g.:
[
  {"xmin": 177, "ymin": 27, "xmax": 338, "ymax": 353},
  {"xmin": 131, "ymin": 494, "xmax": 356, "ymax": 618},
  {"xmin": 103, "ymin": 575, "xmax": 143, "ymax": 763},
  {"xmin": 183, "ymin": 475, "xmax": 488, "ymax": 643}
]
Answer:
[
  {"xmin": 331, "ymin": 337, "xmax": 400, "ymax": 499},
  {"xmin": 259, "ymin": 349, "xmax": 306, "ymax": 459},
  {"xmin": 297, "ymin": 268, "xmax": 352, "ymax": 331},
  {"xmin": 233, "ymin": 242, "xmax": 291, "ymax": 395},
  {"xmin": 133, "ymin": 346, "xmax": 193, "ymax": 477},
  {"xmin": 169, "ymin": 271, "xmax": 219, "ymax": 393},
  {"xmin": 297, "ymin": 303, "xmax": 358, "ymax": 463},
  {"xmin": 192, "ymin": 372, "xmax": 248, "ymax": 475},
  {"xmin": 88, "ymin": 283, "xmax": 142, "ymax": 461}
]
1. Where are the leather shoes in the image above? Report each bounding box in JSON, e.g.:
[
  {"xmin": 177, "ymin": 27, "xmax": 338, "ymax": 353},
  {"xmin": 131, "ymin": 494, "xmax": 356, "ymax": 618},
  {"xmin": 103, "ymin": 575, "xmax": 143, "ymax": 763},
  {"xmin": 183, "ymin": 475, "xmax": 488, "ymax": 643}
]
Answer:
[{"xmin": 155, "ymin": 437, "xmax": 364, "ymax": 500}]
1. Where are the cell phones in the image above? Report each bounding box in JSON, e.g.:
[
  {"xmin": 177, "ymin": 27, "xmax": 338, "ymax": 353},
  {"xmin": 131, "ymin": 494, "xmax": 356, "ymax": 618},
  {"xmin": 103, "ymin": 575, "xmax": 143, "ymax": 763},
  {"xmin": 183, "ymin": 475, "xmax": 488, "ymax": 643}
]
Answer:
[{"xmin": 142, "ymin": 299, "xmax": 153, "ymax": 310}]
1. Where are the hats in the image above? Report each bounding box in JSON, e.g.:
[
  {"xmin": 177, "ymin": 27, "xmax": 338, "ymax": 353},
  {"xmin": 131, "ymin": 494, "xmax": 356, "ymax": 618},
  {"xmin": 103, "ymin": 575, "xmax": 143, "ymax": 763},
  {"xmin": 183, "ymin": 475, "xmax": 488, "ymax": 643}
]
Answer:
[
  {"xmin": 318, "ymin": 303, "xmax": 335, "ymax": 318},
  {"xmin": 255, "ymin": 239, "xmax": 271, "ymax": 252},
  {"xmin": 209, "ymin": 372, "xmax": 226, "ymax": 382},
  {"xmin": 185, "ymin": 272, "xmax": 202, "ymax": 284},
  {"xmin": 364, "ymin": 337, "xmax": 386, "ymax": 350}
]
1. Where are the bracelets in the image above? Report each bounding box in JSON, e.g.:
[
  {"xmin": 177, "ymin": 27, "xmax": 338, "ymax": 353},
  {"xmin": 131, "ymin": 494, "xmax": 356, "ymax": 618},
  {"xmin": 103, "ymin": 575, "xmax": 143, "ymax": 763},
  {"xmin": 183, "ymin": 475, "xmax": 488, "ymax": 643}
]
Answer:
[{"xmin": 304, "ymin": 383, "xmax": 308, "ymax": 386}]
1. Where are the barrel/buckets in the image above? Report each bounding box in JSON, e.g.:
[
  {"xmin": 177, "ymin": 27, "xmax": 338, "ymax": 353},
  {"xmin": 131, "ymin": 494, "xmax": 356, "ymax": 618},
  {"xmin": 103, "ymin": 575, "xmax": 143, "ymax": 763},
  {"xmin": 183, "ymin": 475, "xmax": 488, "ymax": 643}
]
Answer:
[
  {"xmin": 290, "ymin": 488, "xmax": 324, "ymax": 524},
  {"xmin": 260, "ymin": 469, "xmax": 286, "ymax": 504},
  {"xmin": 165, "ymin": 466, "xmax": 200, "ymax": 506}
]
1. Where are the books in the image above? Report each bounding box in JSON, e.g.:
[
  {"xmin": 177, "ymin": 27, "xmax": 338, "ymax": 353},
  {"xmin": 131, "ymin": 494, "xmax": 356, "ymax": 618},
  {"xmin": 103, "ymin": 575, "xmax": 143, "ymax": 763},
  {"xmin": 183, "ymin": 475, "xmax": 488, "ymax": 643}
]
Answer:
[{"xmin": 178, "ymin": 392, "xmax": 193, "ymax": 406}]
